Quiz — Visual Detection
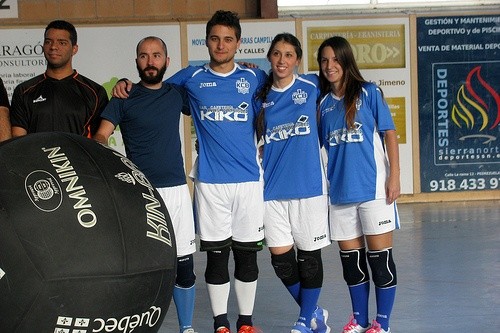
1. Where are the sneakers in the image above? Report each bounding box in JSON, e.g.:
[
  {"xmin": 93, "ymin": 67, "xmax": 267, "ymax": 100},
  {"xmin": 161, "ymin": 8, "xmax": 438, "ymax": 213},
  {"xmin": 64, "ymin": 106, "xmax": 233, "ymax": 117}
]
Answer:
[
  {"xmin": 341, "ymin": 318, "xmax": 371, "ymax": 333},
  {"xmin": 366, "ymin": 317, "xmax": 390, "ymax": 333},
  {"xmin": 290, "ymin": 320, "xmax": 312, "ymax": 333},
  {"xmin": 309, "ymin": 305, "xmax": 331, "ymax": 333}
]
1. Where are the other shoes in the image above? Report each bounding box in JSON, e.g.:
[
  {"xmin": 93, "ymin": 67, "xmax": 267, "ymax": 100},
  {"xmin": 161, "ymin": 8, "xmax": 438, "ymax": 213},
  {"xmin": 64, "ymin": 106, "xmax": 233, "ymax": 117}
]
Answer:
[
  {"xmin": 213, "ymin": 324, "xmax": 229, "ymax": 333},
  {"xmin": 181, "ymin": 328, "xmax": 195, "ymax": 333},
  {"xmin": 238, "ymin": 324, "xmax": 256, "ymax": 333}
]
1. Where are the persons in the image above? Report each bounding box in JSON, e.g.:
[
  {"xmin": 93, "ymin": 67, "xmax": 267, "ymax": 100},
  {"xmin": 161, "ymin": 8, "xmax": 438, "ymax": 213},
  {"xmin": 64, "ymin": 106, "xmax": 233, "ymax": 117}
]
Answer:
[
  {"xmin": 0, "ymin": 76, "xmax": 11, "ymax": 142},
  {"xmin": 110, "ymin": 10, "xmax": 270, "ymax": 333},
  {"xmin": 238, "ymin": 32, "xmax": 331, "ymax": 333},
  {"xmin": 316, "ymin": 35, "xmax": 400, "ymax": 333},
  {"xmin": 93, "ymin": 36, "xmax": 259, "ymax": 333},
  {"xmin": 9, "ymin": 20, "xmax": 109, "ymax": 138}
]
